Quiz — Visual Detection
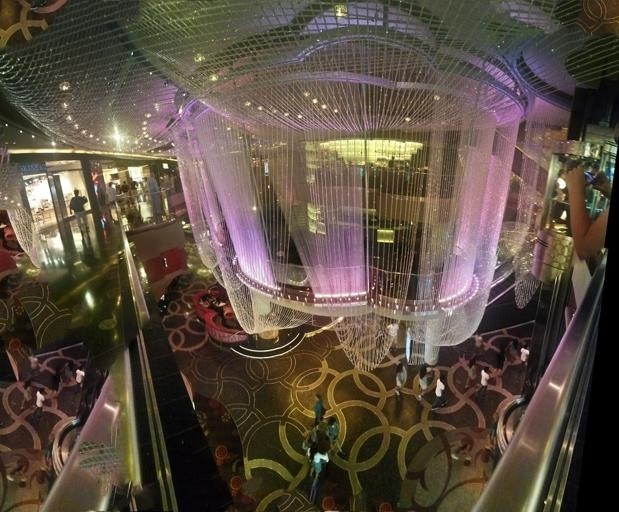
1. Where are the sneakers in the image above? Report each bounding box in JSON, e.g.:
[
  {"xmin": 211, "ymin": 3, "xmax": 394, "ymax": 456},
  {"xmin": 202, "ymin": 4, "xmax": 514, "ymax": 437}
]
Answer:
[{"xmin": 394, "ymin": 389, "xmax": 448, "ymax": 410}]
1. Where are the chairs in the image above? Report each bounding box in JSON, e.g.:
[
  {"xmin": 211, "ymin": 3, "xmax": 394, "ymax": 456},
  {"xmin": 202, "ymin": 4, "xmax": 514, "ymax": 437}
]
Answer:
[{"xmin": 192, "ymin": 290, "xmax": 248, "ymax": 349}]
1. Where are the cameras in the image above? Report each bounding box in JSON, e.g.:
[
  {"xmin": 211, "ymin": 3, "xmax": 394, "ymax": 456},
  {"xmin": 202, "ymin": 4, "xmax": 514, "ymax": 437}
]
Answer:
[{"xmin": 584, "ymin": 172, "xmax": 595, "ymax": 187}]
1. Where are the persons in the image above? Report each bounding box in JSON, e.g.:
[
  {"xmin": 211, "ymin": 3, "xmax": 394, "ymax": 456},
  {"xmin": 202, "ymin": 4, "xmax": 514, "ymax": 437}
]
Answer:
[
  {"xmin": 18, "ymin": 351, "xmax": 85, "ymax": 411},
  {"xmin": 305, "ymin": 333, "xmax": 529, "ymax": 503},
  {"xmin": 388, "ymin": 155, "xmax": 396, "ymax": 168},
  {"xmin": 106, "ymin": 175, "xmax": 149, "ymax": 215},
  {"xmin": 69, "ymin": 189, "xmax": 91, "ymax": 234},
  {"xmin": 560, "ymin": 163, "xmax": 612, "ymax": 260}
]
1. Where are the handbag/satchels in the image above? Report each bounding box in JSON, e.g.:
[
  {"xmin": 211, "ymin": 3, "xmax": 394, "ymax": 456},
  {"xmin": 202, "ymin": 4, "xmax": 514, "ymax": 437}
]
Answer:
[{"xmin": 302, "ymin": 440, "xmax": 312, "ymax": 450}]
